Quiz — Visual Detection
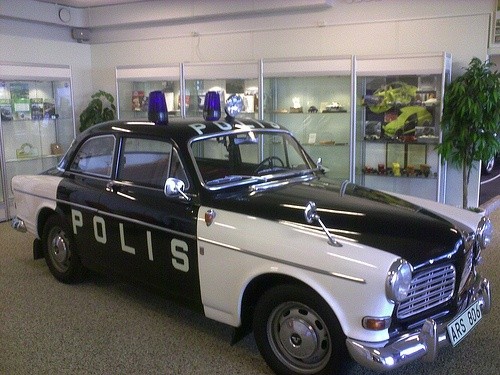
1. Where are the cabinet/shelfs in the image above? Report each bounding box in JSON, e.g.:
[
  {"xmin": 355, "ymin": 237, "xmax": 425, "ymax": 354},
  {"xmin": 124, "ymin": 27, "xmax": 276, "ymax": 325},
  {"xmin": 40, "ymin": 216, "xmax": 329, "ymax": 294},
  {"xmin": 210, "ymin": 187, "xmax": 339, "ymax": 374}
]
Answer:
[
  {"xmin": 115, "ymin": 52, "xmax": 452, "ymax": 205},
  {"xmin": 0, "ymin": 61, "xmax": 77, "ymax": 222}
]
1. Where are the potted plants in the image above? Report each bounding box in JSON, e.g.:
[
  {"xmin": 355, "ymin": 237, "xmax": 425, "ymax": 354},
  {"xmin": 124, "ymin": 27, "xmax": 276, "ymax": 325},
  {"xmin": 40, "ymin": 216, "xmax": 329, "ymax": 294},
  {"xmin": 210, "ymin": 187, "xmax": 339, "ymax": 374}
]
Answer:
[{"xmin": 433, "ymin": 56, "xmax": 500, "ymax": 217}]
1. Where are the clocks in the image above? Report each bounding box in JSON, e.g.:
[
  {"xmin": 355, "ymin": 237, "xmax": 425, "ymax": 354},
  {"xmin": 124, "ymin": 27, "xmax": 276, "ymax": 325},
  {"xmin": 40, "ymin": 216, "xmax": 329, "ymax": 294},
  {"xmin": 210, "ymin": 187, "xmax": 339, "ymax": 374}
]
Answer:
[{"xmin": 16, "ymin": 143, "xmax": 37, "ymax": 158}]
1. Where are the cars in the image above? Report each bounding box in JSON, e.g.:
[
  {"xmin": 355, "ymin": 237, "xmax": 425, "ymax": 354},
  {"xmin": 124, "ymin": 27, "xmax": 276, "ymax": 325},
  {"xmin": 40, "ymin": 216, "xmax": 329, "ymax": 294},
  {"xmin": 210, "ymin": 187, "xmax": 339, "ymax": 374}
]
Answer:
[{"xmin": 9, "ymin": 89, "xmax": 492, "ymax": 375}]
